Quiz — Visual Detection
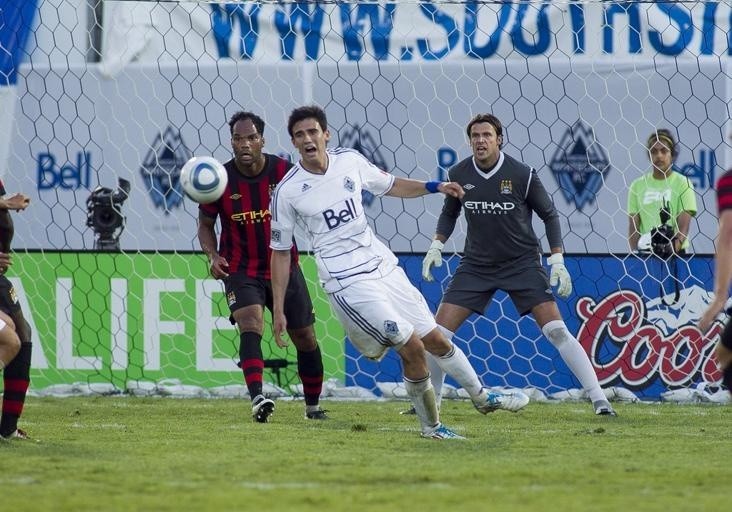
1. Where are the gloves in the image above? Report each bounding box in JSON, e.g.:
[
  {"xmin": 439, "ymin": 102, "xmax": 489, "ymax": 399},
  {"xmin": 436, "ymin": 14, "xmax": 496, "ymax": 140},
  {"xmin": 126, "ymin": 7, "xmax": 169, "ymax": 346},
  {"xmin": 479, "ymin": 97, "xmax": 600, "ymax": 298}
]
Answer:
[
  {"xmin": 422, "ymin": 240, "xmax": 443, "ymax": 283},
  {"xmin": 546, "ymin": 252, "xmax": 573, "ymax": 299}
]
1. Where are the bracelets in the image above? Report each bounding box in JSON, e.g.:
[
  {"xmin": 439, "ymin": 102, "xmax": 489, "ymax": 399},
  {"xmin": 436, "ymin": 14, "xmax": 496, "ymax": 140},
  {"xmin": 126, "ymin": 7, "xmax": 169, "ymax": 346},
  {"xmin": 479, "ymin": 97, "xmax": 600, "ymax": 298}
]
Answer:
[{"xmin": 425, "ymin": 180, "xmax": 440, "ymax": 193}]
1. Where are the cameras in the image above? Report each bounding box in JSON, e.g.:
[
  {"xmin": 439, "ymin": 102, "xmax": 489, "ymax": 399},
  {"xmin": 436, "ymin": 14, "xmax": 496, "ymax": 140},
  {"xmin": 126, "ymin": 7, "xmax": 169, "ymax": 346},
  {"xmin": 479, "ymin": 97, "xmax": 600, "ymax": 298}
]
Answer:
[{"xmin": 651, "ymin": 227, "xmax": 678, "ymax": 258}]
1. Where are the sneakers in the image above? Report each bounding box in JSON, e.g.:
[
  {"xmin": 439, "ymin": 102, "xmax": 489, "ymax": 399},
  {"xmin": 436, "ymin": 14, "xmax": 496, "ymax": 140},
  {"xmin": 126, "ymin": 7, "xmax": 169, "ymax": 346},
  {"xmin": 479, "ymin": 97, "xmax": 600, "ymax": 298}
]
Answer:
[
  {"xmin": 0, "ymin": 424, "xmax": 41, "ymax": 444},
  {"xmin": 250, "ymin": 395, "xmax": 276, "ymax": 423},
  {"xmin": 592, "ymin": 397, "xmax": 617, "ymax": 415},
  {"xmin": 421, "ymin": 422, "xmax": 466, "ymax": 440},
  {"xmin": 471, "ymin": 384, "xmax": 529, "ymax": 415},
  {"xmin": 304, "ymin": 405, "xmax": 334, "ymax": 420}
]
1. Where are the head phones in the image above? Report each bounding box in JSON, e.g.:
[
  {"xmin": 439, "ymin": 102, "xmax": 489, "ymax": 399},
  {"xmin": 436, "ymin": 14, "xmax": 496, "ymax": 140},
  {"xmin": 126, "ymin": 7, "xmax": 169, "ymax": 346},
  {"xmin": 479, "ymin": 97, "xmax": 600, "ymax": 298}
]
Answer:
[{"xmin": 648, "ymin": 135, "xmax": 680, "ymax": 161}]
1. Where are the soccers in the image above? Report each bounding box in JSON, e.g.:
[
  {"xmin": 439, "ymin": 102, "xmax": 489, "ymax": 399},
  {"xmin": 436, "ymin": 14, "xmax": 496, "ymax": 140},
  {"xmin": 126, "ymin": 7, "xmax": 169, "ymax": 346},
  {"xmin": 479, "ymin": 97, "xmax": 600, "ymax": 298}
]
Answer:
[{"xmin": 179, "ymin": 154, "xmax": 229, "ymax": 202}]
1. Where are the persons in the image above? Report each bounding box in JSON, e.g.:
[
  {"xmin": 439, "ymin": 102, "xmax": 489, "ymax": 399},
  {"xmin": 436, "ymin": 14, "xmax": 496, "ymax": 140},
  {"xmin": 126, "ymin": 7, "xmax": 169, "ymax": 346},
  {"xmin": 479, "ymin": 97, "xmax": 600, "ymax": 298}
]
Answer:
[
  {"xmin": 0, "ymin": 312, "xmax": 22, "ymax": 372},
  {"xmin": 399, "ymin": 113, "xmax": 617, "ymax": 416},
  {"xmin": 268, "ymin": 105, "xmax": 530, "ymax": 439},
  {"xmin": 694, "ymin": 168, "xmax": 731, "ymax": 403},
  {"xmin": 0, "ymin": 180, "xmax": 32, "ymax": 442},
  {"xmin": 197, "ymin": 111, "xmax": 329, "ymax": 422},
  {"xmin": 628, "ymin": 128, "xmax": 696, "ymax": 256}
]
